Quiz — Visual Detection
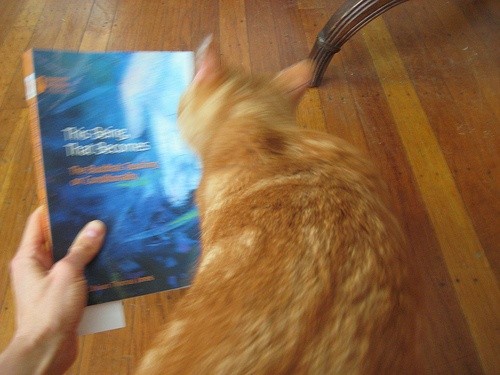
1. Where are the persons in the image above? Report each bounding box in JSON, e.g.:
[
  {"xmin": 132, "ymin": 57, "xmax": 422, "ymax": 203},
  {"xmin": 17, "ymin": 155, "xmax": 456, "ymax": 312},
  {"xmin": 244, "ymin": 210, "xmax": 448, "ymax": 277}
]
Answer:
[{"xmin": 0, "ymin": 206, "xmax": 106, "ymax": 373}]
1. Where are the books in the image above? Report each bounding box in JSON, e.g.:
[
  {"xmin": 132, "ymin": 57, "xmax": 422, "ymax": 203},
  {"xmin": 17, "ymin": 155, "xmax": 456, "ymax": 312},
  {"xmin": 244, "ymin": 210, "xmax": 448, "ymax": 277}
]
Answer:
[{"xmin": 24, "ymin": 48, "xmax": 202, "ymax": 303}]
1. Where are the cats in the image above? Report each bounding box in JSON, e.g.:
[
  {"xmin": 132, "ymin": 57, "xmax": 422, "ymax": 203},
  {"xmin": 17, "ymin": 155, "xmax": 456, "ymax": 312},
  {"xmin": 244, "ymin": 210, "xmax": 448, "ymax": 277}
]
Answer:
[{"xmin": 131, "ymin": 32, "xmax": 409, "ymax": 375}]
